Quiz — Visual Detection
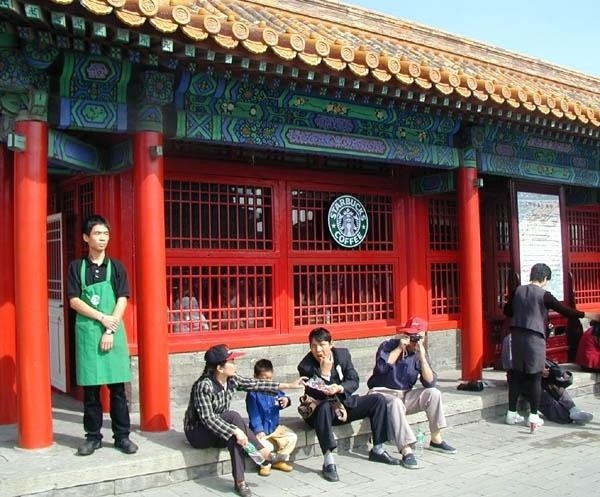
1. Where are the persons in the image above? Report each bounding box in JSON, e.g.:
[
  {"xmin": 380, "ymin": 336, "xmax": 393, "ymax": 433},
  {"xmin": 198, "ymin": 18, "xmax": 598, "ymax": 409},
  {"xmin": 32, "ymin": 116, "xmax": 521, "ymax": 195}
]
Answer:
[
  {"xmin": 337, "ymin": 206, "xmax": 361, "ymax": 237},
  {"xmin": 361, "ymin": 284, "xmax": 381, "ymax": 320},
  {"xmin": 503, "ymin": 331, "xmax": 593, "ymax": 426},
  {"xmin": 576, "ymin": 319, "xmax": 599, "ymax": 373},
  {"xmin": 245, "ymin": 359, "xmax": 296, "ymax": 476},
  {"xmin": 174, "ymin": 287, "xmax": 209, "ymax": 333},
  {"xmin": 65, "ymin": 213, "xmax": 138, "ymax": 456},
  {"xmin": 367, "ymin": 316, "xmax": 457, "ymax": 469},
  {"xmin": 328, "ymin": 286, "xmax": 359, "ymax": 322},
  {"xmin": 183, "ymin": 344, "xmax": 310, "ymax": 497},
  {"xmin": 218, "ymin": 282, "xmax": 262, "ymax": 329},
  {"xmin": 297, "ymin": 326, "xmax": 403, "ymax": 482},
  {"xmin": 300, "ymin": 281, "xmax": 330, "ymax": 325},
  {"xmin": 503, "ymin": 263, "xmax": 599, "ymax": 432}
]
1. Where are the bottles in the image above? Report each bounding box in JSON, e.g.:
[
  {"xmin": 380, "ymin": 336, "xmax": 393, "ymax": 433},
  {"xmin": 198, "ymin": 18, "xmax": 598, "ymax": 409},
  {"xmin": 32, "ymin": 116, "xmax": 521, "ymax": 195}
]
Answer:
[
  {"xmin": 243, "ymin": 441, "xmax": 265, "ymax": 465},
  {"xmin": 415, "ymin": 428, "xmax": 424, "ymax": 457}
]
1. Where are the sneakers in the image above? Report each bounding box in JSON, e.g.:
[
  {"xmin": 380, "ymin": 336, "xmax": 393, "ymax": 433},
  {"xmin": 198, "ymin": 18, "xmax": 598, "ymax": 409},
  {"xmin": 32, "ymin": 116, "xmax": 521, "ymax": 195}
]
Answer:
[
  {"xmin": 77, "ymin": 433, "xmax": 103, "ymax": 457},
  {"xmin": 526, "ymin": 412, "xmax": 544, "ymax": 426},
  {"xmin": 505, "ymin": 408, "xmax": 527, "ymax": 427},
  {"xmin": 112, "ymin": 434, "xmax": 139, "ymax": 454},
  {"xmin": 271, "ymin": 459, "xmax": 295, "ymax": 474},
  {"xmin": 456, "ymin": 379, "xmax": 485, "ymax": 391},
  {"xmin": 258, "ymin": 463, "xmax": 271, "ymax": 477},
  {"xmin": 429, "ymin": 439, "xmax": 456, "ymax": 456},
  {"xmin": 400, "ymin": 453, "xmax": 419, "ymax": 469}
]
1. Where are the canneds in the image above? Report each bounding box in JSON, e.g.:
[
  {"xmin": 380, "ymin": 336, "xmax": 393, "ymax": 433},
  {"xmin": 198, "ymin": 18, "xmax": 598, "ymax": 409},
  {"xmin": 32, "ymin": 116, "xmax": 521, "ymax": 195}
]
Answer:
[{"xmin": 531, "ymin": 422, "xmax": 537, "ymax": 434}]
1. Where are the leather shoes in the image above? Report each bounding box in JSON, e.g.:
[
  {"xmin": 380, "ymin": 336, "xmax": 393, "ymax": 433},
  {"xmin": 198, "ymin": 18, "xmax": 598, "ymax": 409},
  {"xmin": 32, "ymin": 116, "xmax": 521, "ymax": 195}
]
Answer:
[
  {"xmin": 233, "ymin": 480, "xmax": 251, "ymax": 497},
  {"xmin": 264, "ymin": 450, "xmax": 288, "ymax": 464},
  {"xmin": 321, "ymin": 462, "xmax": 341, "ymax": 484},
  {"xmin": 368, "ymin": 448, "xmax": 398, "ymax": 467}
]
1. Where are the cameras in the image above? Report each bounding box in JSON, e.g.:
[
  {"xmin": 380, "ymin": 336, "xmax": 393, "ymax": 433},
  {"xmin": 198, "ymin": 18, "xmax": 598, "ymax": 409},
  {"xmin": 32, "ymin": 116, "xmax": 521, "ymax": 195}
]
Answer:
[{"xmin": 410, "ymin": 335, "xmax": 423, "ymax": 342}]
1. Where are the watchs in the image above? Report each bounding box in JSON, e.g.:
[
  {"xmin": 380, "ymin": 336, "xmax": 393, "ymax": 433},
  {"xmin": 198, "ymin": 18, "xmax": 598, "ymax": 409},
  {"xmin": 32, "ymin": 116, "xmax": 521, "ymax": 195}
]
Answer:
[{"xmin": 104, "ymin": 328, "xmax": 113, "ymax": 336}]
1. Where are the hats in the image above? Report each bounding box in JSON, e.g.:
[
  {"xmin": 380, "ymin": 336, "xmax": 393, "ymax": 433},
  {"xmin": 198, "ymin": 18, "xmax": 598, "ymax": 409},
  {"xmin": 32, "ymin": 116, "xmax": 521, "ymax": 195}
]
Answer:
[
  {"xmin": 394, "ymin": 315, "xmax": 430, "ymax": 338},
  {"xmin": 204, "ymin": 343, "xmax": 249, "ymax": 363}
]
